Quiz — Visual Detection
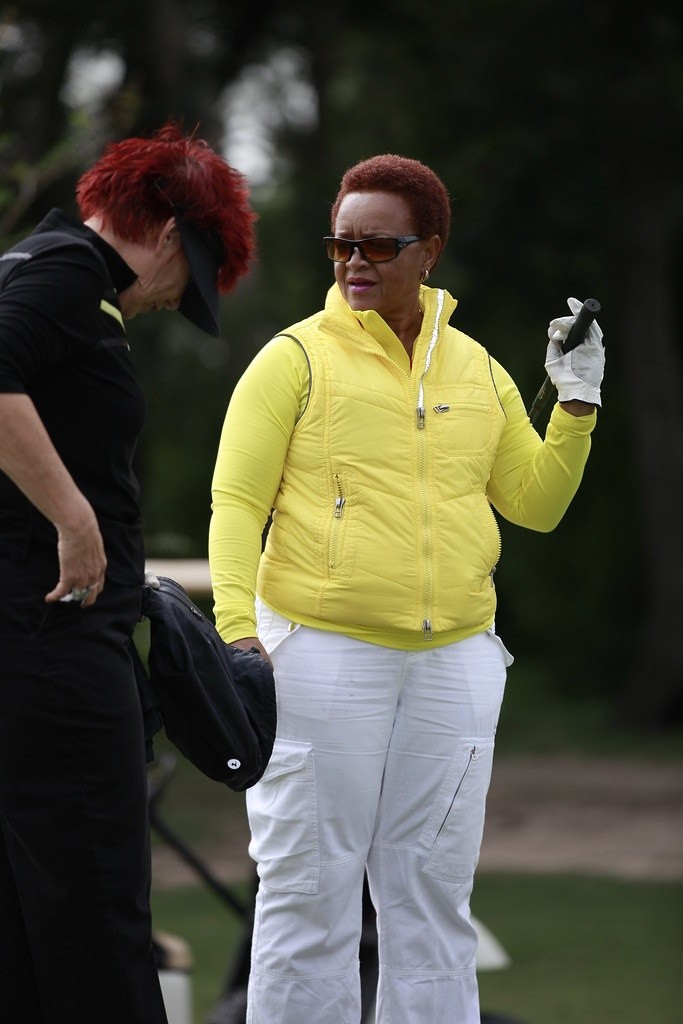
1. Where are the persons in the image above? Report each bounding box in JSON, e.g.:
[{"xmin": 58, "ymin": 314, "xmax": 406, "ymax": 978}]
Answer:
[
  {"xmin": 0, "ymin": 117, "xmax": 258, "ymax": 1024},
  {"xmin": 205, "ymin": 148, "xmax": 608, "ymax": 1024}
]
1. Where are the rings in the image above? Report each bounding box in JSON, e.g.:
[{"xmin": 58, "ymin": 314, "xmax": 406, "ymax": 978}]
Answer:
[
  {"xmin": 72, "ymin": 584, "xmax": 87, "ymax": 599},
  {"xmin": 88, "ymin": 580, "xmax": 100, "ymax": 592}
]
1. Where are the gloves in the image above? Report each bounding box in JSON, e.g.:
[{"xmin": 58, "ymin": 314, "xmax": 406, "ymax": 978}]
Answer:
[{"xmin": 546, "ymin": 298, "xmax": 604, "ymax": 407}]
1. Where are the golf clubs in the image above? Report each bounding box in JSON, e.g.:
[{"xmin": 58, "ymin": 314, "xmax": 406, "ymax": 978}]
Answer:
[
  {"xmin": 150, "ymin": 825, "xmax": 506, "ymax": 1023},
  {"xmin": 524, "ymin": 299, "xmax": 599, "ymax": 426}
]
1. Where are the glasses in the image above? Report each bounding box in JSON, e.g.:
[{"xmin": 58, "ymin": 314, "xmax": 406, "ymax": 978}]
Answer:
[{"xmin": 323, "ymin": 233, "xmax": 421, "ymax": 262}]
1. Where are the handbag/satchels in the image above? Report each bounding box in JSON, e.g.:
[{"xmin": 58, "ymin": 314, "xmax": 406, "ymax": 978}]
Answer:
[{"xmin": 132, "ymin": 574, "xmax": 276, "ymax": 792}]
